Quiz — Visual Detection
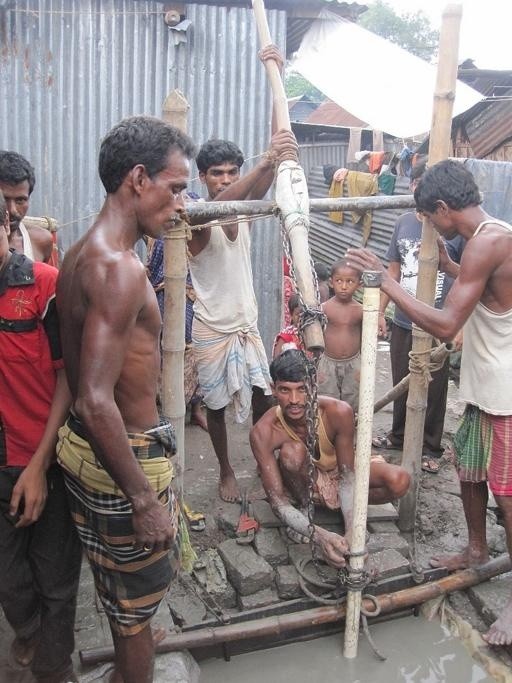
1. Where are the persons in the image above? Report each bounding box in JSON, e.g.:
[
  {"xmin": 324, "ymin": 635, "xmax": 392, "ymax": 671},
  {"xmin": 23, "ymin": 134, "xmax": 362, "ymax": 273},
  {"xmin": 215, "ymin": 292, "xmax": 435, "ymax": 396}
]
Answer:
[
  {"xmin": 0, "ymin": 151, "xmax": 53, "ymax": 266},
  {"xmin": 250, "ymin": 351, "xmax": 411, "ymax": 568},
  {"xmin": 316, "ymin": 258, "xmax": 363, "ymax": 449},
  {"xmin": 341, "ymin": 161, "xmax": 512, "ymax": 647},
  {"xmin": 274, "ymin": 296, "xmax": 301, "ymax": 359},
  {"xmin": 182, "ymin": 128, "xmax": 299, "ymax": 503},
  {"xmin": 139, "ymin": 193, "xmax": 208, "ymax": 429},
  {"xmin": 55, "ymin": 115, "xmax": 197, "ymax": 683},
  {"xmin": 372, "ymin": 164, "xmax": 468, "ymax": 472},
  {"xmin": 0, "ymin": 193, "xmax": 83, "ymax": 683}
]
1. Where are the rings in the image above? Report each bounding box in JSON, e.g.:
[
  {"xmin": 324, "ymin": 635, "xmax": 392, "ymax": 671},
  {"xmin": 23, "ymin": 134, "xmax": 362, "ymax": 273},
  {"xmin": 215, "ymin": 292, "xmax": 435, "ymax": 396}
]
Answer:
[{"xmin": 144, "ymin": 546, "xmax": 151, "ymax": 552}]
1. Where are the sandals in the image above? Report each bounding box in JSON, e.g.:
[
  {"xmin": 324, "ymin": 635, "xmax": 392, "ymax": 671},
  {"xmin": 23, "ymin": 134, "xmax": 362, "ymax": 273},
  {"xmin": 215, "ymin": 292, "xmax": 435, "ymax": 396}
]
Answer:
[
  {"xmin": 422, "ymin": 456, "xmax": 440, "ymax": 473},
  {"xmin": 372, "ymin": 436, "xmax": 396, "ymax": 449}
]
